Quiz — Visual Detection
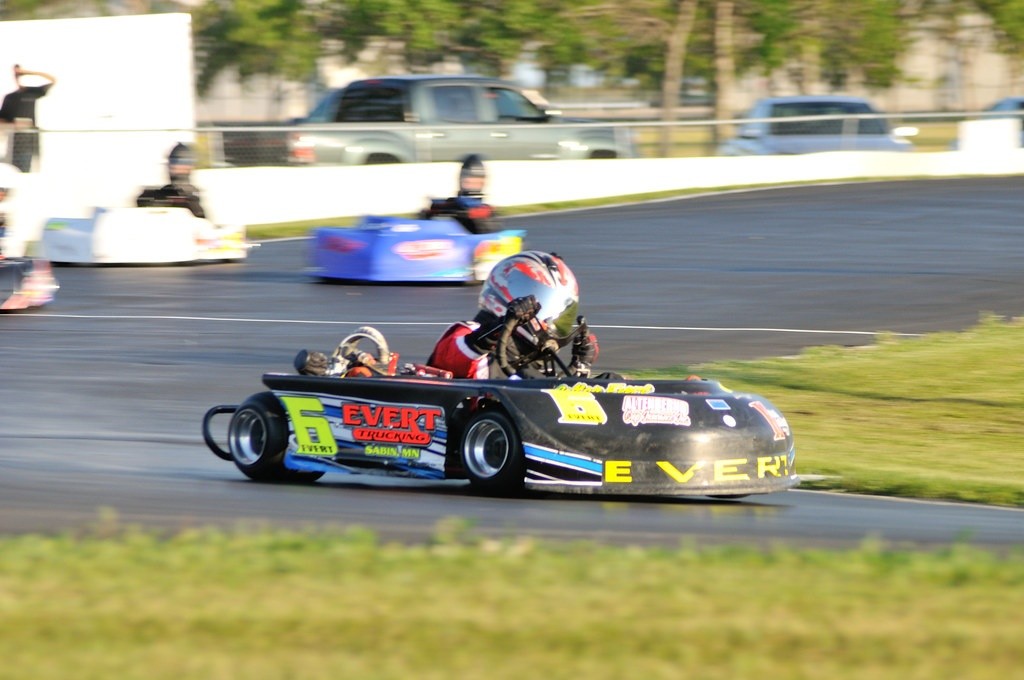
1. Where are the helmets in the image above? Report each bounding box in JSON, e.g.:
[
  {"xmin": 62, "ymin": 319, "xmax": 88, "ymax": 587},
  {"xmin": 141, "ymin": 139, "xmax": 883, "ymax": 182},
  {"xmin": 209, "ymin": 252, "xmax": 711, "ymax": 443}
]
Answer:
[
  {"xmin": 478, "ymin": 251, "xmax": 579, "ymax": 355},
  {"xmin": 167, "ymin": 144, "xmax": 195, "ymax": 188},
  {"xmin": 458, "ymin": 155, "xmax": 486, "ymax": 199}
]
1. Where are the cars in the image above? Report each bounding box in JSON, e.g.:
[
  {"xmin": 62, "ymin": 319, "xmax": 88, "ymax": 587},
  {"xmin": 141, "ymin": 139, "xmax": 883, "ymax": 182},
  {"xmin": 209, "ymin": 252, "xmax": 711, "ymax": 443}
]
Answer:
[
  {"xmin": 716, "ymin": 95, "xmax": 913, "ymax": 152},
  {"xmin": 290, "ymin": 77, "xmax": 631, "ymax": 162}
]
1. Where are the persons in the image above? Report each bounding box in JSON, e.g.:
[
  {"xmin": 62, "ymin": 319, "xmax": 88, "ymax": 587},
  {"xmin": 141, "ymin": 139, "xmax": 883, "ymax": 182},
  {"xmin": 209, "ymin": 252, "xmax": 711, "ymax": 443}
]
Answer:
[
  {"xmin": 417, "ymin": 153, "xmax": 502, "ymax": 233},
  {"xmin": 136, "ymin": 142, "xmax": 204, "ymax": 217},
  {"xmin": 0, "ymin": 64, "xmax": 55, "ymax": 173},
  {"xmin": 425, "ymin": 249, "xmax": 598, "ymax": 381}
]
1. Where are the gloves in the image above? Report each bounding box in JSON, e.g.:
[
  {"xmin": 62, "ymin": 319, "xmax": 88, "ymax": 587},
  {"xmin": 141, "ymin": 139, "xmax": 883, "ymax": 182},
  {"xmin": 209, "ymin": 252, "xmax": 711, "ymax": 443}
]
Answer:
[
  {"xmin": 504, "ymin": 295, "xmax": 541, "ymax": 327},
  {"xmin": 571, "ymin": 334, "xmax": 599, "ymax": 363}
]
